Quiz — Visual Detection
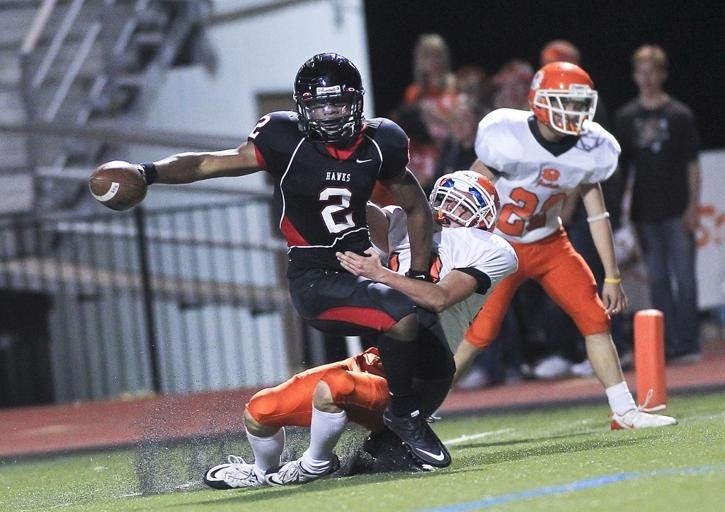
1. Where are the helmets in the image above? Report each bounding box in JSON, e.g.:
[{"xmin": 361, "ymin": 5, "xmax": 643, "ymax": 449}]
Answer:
[
  {"xmin": 527, "ymin": 61, "xmax": 599, "ymax": 138},
  {"xmin": 429, "ymin": 170, "xmax": 500, "ymax": 232},
  {"xmin": 292, "ymin": 52, "xmax": 364, "ymax": 149}
]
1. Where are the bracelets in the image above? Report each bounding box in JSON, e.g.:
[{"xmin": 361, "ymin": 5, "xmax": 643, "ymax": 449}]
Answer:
[{"xmin": 141, "ymin": 163, "xmax": 158, "ymax": 185}]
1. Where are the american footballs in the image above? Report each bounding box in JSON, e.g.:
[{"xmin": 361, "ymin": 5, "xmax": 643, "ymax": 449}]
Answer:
[{"xmin": 88, "ymin": 160, "xmax": 148, "ymax": 211}]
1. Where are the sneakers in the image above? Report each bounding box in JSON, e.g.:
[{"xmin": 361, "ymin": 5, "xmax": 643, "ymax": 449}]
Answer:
[
  {"xmin": 362, "ymin": 431, "xmax": 436, "ymax": 472},
  {"xmin": 204, "ymin": 462, "xmax": 268, "ymax": 490},
  {"xmin": 611, "ymin": 407, "xmax": 678, "ymax": 430},
  {"xmin": 381, "ymin": 406, "xmax": 451, "ymax": 468},
  {"xmin": 265, "ymin": 453, "xmax": 340, "ymax": 486}
]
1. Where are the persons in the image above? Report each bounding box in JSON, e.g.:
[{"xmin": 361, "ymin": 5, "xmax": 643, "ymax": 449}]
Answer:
[
  {"xmin": 432, "ymin": 58, "xmax": 680, "ymax": 431},
  {"xmin": 200, "ymin": 167, "xmax": 520, "ymax": 493},
  {"xmin": 365, "ymin": 28, "xmax": 703, "ymax": 392},
  {"xmin": 131, "ymin": 51, "xmax": 456, "ymax": 474}
]
